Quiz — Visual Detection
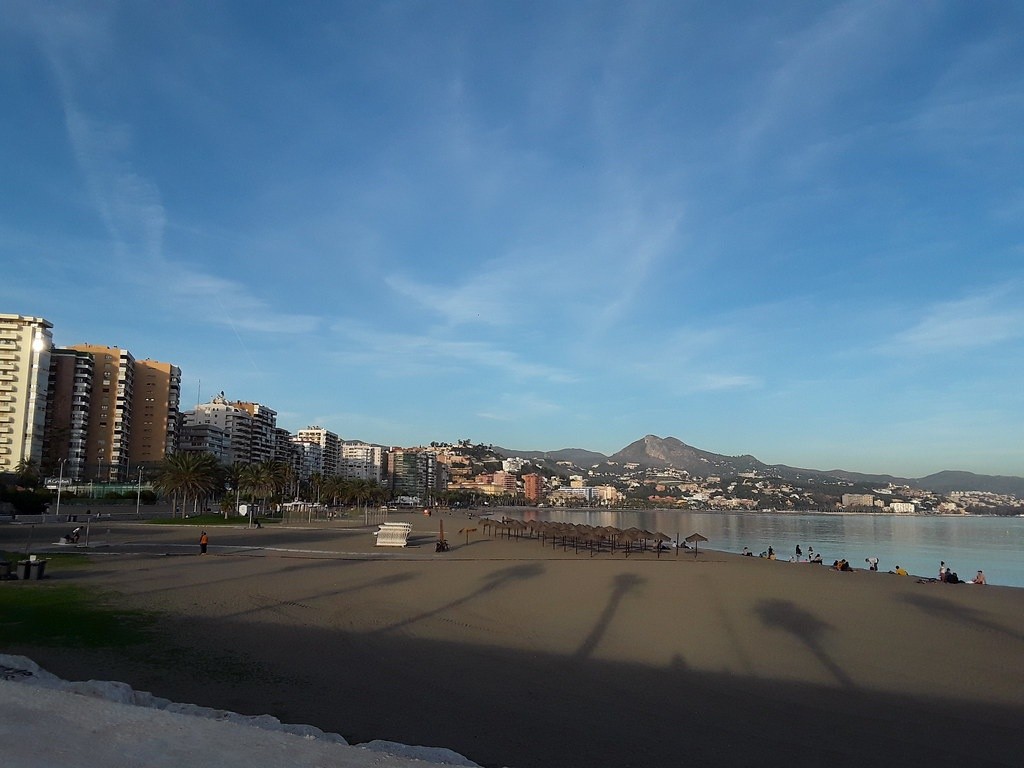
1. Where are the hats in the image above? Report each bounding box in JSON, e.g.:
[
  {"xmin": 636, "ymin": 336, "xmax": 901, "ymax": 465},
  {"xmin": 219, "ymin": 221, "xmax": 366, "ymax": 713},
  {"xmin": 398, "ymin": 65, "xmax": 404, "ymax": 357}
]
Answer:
[{"xmin": 977, "ymin": 570, "xmax": 982, "ymax": 573}]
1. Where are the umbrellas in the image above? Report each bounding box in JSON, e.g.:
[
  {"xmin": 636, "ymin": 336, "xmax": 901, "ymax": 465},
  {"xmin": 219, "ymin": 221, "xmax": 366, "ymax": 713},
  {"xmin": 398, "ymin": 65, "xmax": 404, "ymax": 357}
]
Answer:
[
  {"xmin": 686, "ymin": 533, "xmax": 708, "ymax": 556},
  {"xmin": 478, "ymin": 518, "xmax": 671, "ymax": 558},
  {"xmin": 457, "ymin": 527, "xmax": 476, "ymax": 544}
]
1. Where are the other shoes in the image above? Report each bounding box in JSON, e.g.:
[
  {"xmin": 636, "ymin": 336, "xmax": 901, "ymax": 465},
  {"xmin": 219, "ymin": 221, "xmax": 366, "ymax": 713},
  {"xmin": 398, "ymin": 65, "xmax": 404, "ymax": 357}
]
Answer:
[{"xmin": 201, "ymin": 553, "xmax": 206, "ymax": 555}]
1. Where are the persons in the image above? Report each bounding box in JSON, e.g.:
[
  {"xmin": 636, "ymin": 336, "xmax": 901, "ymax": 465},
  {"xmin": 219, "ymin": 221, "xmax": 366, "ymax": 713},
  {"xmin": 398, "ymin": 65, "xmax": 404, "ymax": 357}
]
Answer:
[
  {"xmin": 974, "ymin": 570, "xmax": 986, "ymax": 585},
  {"xmin": 200, "ymin": 531, "xmax": 208, "ymax": 556},
  {"xmin": 938, "ymin": 561, "xmax": 959, "ymax": 584},
  {"xmin": 72, "ymin": 525, "xmax": 83, "ymax": 543},
  {"xmin": 865, "ymin": 558, "xmax": 879, "ymax": 571},
  {"xmin": 833, "ymin": 559, "xmax": 853, "ymax": 572},
  {"xmin": 743, "ymin": 547, "xmax": 753, "ymax": 556},
  {"xmin": 767, "ymin": 546, "xmax": 774, "ymax": 559},
  {"xmin": 423, "ymin": 508, "xmax": 432, "ymax": 520},
  {"xmin": 107, "ymin": 512, "xmax": 111, "ymax": 520},
  {"xmin": 789, "ymin": 544, "xmax": 822, "ymax": 565},
  {"xmin": 652, "ymin": 540, "xmax": 693, "ymax": 550},
  {"xmin": 896, "ymin": 566, "xmax": 908, "ymax": 577},
  {"xmin": 95, "ymin": 512, "xmax": 100, "ymax": 523}
]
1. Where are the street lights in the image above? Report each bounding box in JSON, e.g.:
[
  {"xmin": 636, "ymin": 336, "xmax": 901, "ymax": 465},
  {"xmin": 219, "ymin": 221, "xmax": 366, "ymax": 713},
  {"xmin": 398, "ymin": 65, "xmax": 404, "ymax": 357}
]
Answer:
[
  {"xmin": 97, "ymin": 457, "xmax": 104, "ymax": 478},
  {"xmin": 56, "ymin": 458, "xmax": 67, "ymax": 514},
  {"xmin": 137, "ymin": 465, "xmax": 145, "ymax": 513}
]
